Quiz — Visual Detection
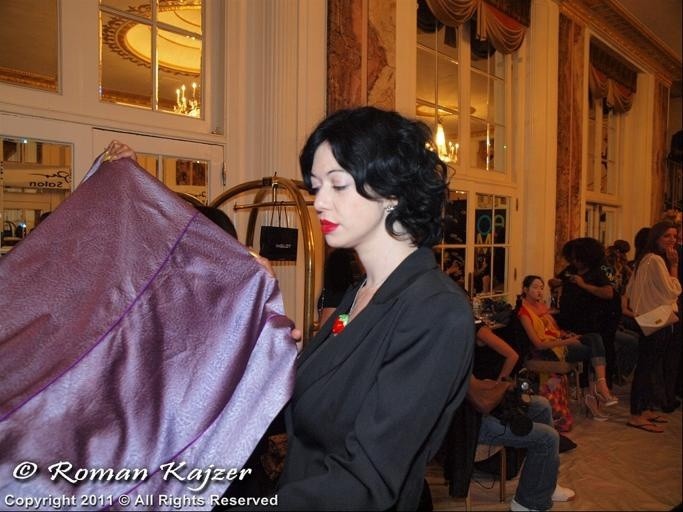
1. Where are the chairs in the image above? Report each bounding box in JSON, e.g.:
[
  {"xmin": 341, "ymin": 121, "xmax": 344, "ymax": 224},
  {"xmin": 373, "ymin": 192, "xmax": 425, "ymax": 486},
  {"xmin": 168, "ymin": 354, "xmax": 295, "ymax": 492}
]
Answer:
[{"xmin": 510, "ymin": 292, "xmax": 588, "ymax": 417}]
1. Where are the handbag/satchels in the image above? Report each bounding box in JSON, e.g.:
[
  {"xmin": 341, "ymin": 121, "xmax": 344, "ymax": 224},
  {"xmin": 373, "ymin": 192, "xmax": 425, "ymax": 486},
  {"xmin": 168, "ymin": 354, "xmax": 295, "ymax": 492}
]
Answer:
[{"xmin": 634, "ymin": 304, "xmax": 680, "ymax": 337}]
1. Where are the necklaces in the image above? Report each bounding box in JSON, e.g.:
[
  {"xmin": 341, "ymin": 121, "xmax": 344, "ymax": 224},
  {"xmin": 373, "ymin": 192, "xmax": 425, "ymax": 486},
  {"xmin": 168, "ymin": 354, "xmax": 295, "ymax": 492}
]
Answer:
[{"xmin": 332, "ymin": 273, "xmax": 388, "ymax": 338}]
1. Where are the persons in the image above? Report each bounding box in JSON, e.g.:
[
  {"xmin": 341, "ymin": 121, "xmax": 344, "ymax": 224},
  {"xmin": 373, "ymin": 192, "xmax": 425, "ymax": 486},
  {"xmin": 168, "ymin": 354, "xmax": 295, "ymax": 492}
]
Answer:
[
  {"xmin": 513, "ymin": 210, "xmax": 683, "ymax": 438},
  {"xmin": 443, "ymin": 365, "xmax": 578, "ymax": 512},
  {"xmin": 430, "ymin": 224, "xmax": 505, "ymax": 294},
  {"xmin": 211, "ymin": 112, "xmax": 478, "ymax": 512},
  {"xmin": 100, "ymin": 138, "xmax": 239, "ymax": 241},
  {"xmin": 460, "ymin": 284, "xmax": 520, "ymax": 383}
]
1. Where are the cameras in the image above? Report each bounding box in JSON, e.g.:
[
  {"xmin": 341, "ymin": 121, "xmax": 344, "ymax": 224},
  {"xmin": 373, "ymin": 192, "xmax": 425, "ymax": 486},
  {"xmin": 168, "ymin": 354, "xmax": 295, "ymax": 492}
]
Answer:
[{"xmin": 494, "ymin": 378, "xmax": 534, "ymax": 437}]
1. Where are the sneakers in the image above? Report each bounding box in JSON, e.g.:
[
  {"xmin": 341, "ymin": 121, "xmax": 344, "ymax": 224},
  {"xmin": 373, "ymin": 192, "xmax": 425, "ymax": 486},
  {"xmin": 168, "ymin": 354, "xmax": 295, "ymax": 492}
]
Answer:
[
  {"xmin": 650, "ymin": 395, "xmax": 682, "ymax": 414},
  {"xmin": 510, "ymin": 495, "xmax": 550, "ymax": 512},
  {"xmin": 551, "ymin": 483, "xmax": 576, "ymax": 502}
]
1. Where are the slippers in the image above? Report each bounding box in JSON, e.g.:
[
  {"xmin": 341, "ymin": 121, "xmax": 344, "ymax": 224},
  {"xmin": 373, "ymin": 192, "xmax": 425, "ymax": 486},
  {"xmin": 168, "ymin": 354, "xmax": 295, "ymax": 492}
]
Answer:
[
  {"xmin": 646, "ymin": 415, "xmax": 669, "ymax": 424},
  {"xmin": 626, "ymin": 422, "xmax": 664, "ymax": 434}
]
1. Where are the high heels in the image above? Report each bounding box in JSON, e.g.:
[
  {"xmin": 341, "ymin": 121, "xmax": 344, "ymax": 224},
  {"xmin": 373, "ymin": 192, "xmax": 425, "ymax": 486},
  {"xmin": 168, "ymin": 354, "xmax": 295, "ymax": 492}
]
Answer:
[
  {"xmin": 594, "ymin": 378, "xmax": 618, "ymax": 407},
  {"xmin": 583, "ymin": 393, "xmax": 609, "ymax": 422}
]
